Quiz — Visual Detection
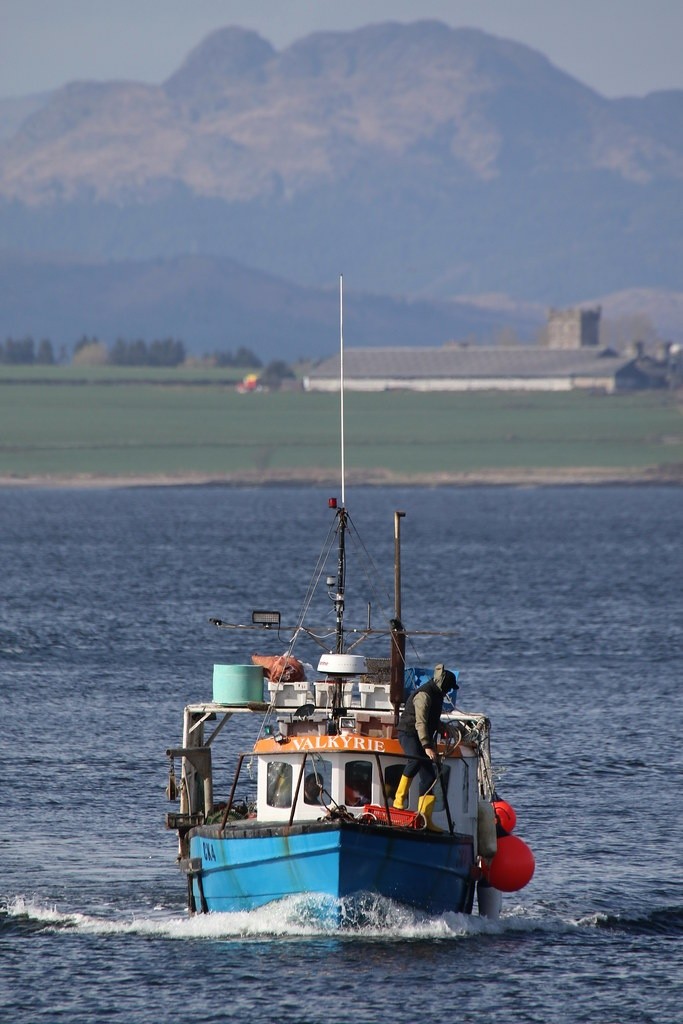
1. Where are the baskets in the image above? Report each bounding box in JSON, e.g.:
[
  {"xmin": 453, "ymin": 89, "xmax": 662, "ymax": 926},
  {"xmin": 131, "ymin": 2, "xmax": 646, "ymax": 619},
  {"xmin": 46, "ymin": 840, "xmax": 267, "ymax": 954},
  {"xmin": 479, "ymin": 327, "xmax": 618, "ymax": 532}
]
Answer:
[{"xmin": 363, "ymin": 804, "xmax": 421, "ymax": 828}]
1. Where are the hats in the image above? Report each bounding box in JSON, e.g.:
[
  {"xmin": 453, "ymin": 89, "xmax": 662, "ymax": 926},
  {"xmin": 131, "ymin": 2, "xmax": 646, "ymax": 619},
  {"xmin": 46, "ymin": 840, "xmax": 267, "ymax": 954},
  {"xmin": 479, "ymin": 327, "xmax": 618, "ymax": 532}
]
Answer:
[{"xmin": 444, "ymin": 669, "xmax": 460, "ymax": 690}]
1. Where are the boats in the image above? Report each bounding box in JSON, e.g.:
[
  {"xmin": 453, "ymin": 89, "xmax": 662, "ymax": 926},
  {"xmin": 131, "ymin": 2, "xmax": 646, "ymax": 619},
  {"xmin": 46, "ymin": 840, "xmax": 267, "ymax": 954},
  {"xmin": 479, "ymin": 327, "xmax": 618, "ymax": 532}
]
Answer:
[{"xmin": 164, "ymin": 274, "xmax": 534, "ymax": 936}]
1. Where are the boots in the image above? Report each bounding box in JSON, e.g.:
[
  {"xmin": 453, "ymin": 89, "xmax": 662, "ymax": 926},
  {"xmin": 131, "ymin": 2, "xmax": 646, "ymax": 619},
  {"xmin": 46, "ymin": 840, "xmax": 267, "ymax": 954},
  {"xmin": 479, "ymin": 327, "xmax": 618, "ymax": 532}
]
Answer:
[
  {"xmin": 392, "ymin": 776, "xmax": 413, "ymax": 810},
  {"xmin": 416, "ymin": 795, "xmax": 444, "ymax": 832}
]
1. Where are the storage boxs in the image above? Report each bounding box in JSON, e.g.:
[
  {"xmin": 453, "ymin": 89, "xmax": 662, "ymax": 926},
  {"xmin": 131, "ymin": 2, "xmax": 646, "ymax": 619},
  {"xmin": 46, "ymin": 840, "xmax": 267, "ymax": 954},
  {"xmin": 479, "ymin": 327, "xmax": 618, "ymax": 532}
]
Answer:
[
  {"xmin": 363, "ymin": 804, "xmax": 421, "ymax": 828},
  {"xmin": 268, "ymin": 681, "xmax": 310, "ymax": 707},
  {"xmin": 312, "ymin": 682, "xmax": 353, "ymax": 708},
  {"xmin": 357, "ymin": 682, "xmax": 394, "ymax": 709}
]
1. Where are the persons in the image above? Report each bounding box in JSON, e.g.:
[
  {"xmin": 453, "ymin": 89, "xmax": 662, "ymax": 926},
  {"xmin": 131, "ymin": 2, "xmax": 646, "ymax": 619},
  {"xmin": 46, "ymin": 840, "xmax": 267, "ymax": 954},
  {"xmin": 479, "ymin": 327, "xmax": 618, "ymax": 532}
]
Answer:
[
  {"xmin": 393, "ymin": 670, "xmax": 459, "ymax": 833},
  {"xmin": 471, "ymin": 801, "xmax": 516, "ymax": 919},
  {"xmin": 304, "ymin": 772, "xmax": 408, "ymax": 810}
]
